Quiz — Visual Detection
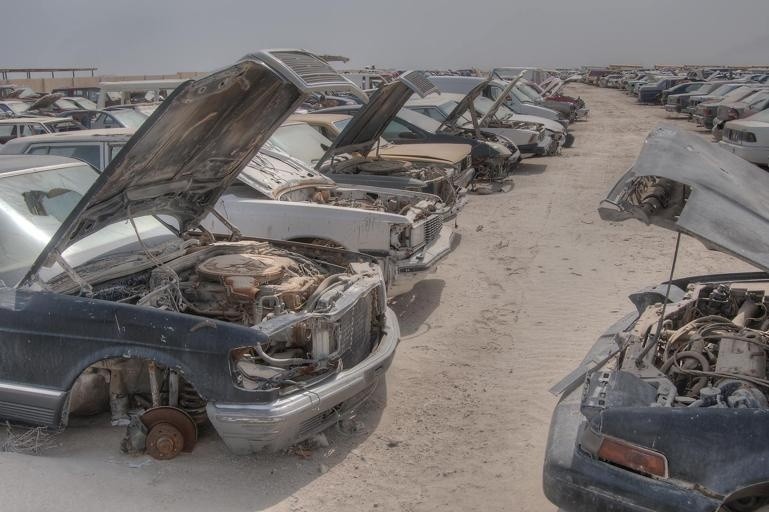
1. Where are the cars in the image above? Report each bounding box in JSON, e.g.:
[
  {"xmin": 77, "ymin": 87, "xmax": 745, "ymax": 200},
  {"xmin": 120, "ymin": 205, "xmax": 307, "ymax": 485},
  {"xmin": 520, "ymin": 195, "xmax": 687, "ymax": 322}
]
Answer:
[
  {"xmin": 0, "ymin": 49, "xmax": 401, "ymax": 462},
  {"xmin": 541, "ymin": 117, "xmax": 768, "ymax": 511},
  {"xmin": 266, "ymin": 69, "xmax": 470, "ymax": 219},
  {"xmin": 0, "ymin": 68, "xmax": 769, "ymax": 158},
  {"xmin": 281, "ymin": 107, "xmax": 479, "ymax": 192},
  {"xmin": 308, "ymin": 76, "xmax": 521, "ymax": 181},
  {"xmin": 718, "ymin": 109, "xmax": 769, "ymax": 166},
  {"xmin": 1, "ymin": 128, "xmax": 467, "ymax": 295}
]
